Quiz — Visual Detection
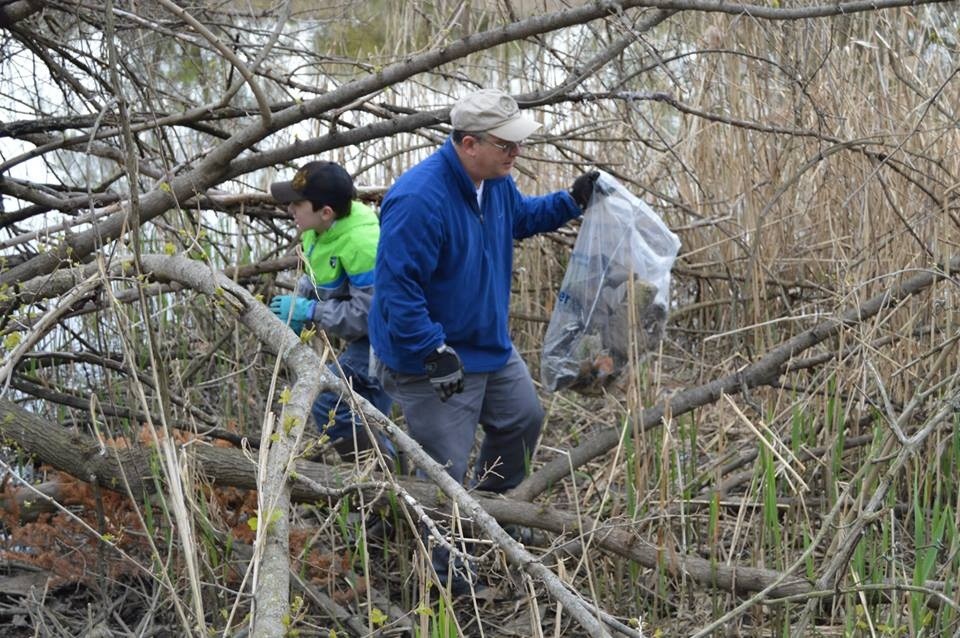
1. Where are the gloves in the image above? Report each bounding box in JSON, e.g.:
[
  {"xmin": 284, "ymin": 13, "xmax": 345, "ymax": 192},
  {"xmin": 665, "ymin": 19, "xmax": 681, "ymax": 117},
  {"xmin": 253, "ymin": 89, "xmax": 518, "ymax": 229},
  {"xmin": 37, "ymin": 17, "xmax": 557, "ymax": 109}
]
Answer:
[
  {"xmin": 568, "ymin": 171, "xmax": 616, "ymax": 209},
  {"xmin": 271, "ymin": 295, "xmax": 316, "ymax": 322},
  {"xmin": 424, "ymin": 345, "xmax": 464, "ymax": 401}
]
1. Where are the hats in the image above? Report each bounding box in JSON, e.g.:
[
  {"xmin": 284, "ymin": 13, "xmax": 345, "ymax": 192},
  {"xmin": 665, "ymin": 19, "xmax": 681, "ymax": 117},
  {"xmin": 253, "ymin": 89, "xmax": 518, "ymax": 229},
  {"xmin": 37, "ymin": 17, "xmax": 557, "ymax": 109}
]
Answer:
[
  {"xmin": 268, "ymin": 160, "xmax": 354, "ymax": 202},
  {"xmin": 450, "ymin": 88, "xmax": 544, "ymax": 143}
]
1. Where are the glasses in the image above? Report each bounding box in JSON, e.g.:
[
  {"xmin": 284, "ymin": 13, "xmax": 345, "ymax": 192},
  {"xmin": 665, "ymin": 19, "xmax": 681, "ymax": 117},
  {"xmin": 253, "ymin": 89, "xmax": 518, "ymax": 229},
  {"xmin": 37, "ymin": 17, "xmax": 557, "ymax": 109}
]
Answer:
[{"xmin": 463, "ymin": 133, "xmax": 511, "ymax": 152}]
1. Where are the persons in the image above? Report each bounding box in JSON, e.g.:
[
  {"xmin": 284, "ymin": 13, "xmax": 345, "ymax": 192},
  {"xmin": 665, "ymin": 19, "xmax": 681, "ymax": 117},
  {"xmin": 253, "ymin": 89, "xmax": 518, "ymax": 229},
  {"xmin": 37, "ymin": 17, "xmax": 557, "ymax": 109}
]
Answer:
[
  {"xmin": 367, "ymin": 87, "xmax": 616, "ymax": 604},
  {"xmin": 269, "ymin": 160, "xmax": 396, "ymax": 483}
]
